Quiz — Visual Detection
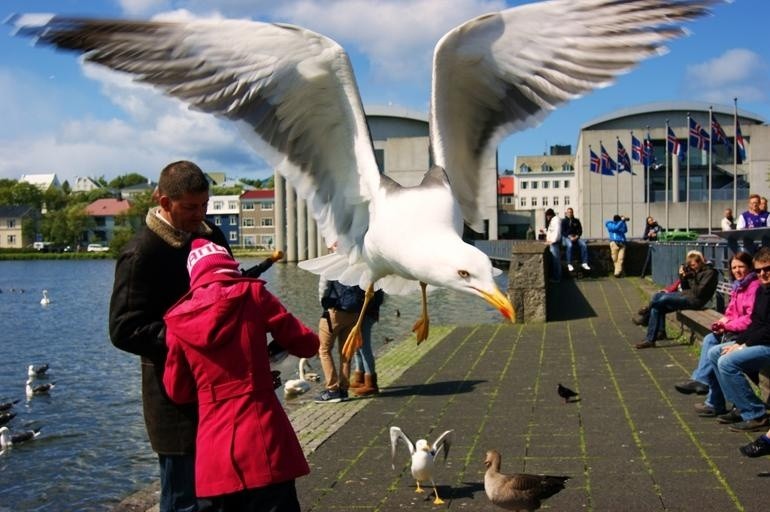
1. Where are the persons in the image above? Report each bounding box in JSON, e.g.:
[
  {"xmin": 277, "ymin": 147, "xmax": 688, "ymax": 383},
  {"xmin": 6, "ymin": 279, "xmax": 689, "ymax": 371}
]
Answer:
[
  {"xmin": 317, "ymin": 241, "xmax": 361, "ymax": 402},
  {"xmin": 643, "ymin": 216, "xmax": 663, "ymax": 241},
  {"xmin": 631, "ymin": 250, "xmax": 770, "ymax": 456},
  {"xmin": 604, "ymin": 214, "xmax": 628, "ymax": 278},
  {"xmin": 720, "ymin": 194, "xmax": 770, "ymax": 231},
  {"xmin": 526, "ymin": 208, "xmax": 591, "ymax": 284},
  {"xmin": 110, "ymin": 161, "xmax": 236, "ymax": 512},
  {"xmin": 348, "ymin": 289, "xmax": 384, "ymax": 396},
  {"xmin": 163, "ymin": 238, "xmax": 319, "ymax": 512}
]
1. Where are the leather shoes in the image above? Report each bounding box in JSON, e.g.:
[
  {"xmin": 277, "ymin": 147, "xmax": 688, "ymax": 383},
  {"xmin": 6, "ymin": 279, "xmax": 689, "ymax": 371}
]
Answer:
[{"xmin": 632, "ymin": 306, "xmax": 667, "ymax": 349}]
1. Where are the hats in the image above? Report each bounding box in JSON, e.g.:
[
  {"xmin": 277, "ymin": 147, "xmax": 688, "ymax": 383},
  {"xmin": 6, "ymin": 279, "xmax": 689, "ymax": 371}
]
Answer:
[{"xmin": 186, "ymin": 239, "xmax": 242, "ymax": 290}]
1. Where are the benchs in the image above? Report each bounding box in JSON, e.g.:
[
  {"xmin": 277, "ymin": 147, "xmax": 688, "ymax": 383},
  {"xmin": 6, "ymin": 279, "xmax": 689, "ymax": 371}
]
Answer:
[{"xmin": 675, "ymin": 279, "xmax": 770, "ymax": 408}]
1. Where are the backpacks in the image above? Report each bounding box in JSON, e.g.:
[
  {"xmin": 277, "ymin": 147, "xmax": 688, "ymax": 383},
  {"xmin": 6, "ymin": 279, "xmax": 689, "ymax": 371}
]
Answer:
[{"xmin": 322, "ymin": 279, "xmax": 360, "ymax": 313}]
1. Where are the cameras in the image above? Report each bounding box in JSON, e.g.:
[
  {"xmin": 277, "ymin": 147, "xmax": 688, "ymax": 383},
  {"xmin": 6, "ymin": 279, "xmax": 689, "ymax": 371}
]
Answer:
[{"xmin": 624, "ymin": 218, "xmax": 629, "ymax": 221}]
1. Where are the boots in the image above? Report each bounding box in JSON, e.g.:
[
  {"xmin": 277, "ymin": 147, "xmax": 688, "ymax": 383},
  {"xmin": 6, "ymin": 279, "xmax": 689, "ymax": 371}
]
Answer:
[
  {"xmin": 353, "ymin": 373, "xmax": 380, "ymax": 397},
  {"xmin": 348, "ymin": 370, "xmax": 364, "ymax": 389}
]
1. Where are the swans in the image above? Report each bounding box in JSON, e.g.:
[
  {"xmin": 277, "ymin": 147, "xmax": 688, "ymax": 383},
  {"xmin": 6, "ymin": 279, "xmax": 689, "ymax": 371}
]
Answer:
[
  {"xmin": 283, "ymin": 357, "xmax": 313, "ymax": 395},
  {"xmin": 39, "ymin": 289, "xmax": 51, "ymax": 305},
  {"xmin": 297, "ymin": 369, "xmax": 321, "ymax": 383}
]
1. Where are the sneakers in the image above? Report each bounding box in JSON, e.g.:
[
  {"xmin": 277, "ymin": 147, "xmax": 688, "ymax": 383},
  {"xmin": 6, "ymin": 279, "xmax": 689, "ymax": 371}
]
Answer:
[
  {"xmin": 314, "ymin": 390, "xmax": 343, "ymax": 403},
  {"xmin": 693, "ymin": 402, "xmax": 727, "ymax": 414},
  {"xmin": 582, "ymin": 263, "xmax": 590, "ymax": 270},
  {"xmin": 567, "ymin": 264, "xmax": 575, "ymax": 272},
  {"xmin": 717, "ymin": 411, "xmax": 743, "ymax": 422},
  {"xmin": 728, "ymin": 418, "xmax": 768, "ymax": 432},
  {"xmin": 674, "ymin": 380, "xmax": 709, "ymax": 395},
  {"xmin": 342, "ymin": 390, "xmax": 349, "ymax": 401},
  {"xmin": 739, "ymin": 434, "xmax": 770, "ymax": 458}
]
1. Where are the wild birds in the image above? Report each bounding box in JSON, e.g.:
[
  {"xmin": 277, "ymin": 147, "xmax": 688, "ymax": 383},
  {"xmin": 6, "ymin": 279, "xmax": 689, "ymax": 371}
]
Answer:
[
  {"xmin": 389, "ymin": 426, "xmax": 455, "ymax": 505},
  {"xmin": 27, "ymin": 363, "xmax": 50, "ymax": 376},
  {"xmin": 483, "ymin": 449, "xmax": 575, "ymax": 512},
  {"xmin": 0, "ymin": 399, "xmax": 21, "ymax": 411},
  {"xmin": 0, "ymin": 0, "xmax": 737, "ymax": 367},
  {"xmin": 25, "ymin": 379, "xmax": 57, "ymax": 397},
  {"xmin": 557, "ymin": 384, "xmax": 578, "ymax": 404},
  {"xmin": 0, "ymin": 412, "xmax": 18, "ymax": 426},
  {"xmin": 0, "ymin": 424, "xmax": 47, "ymax": 450}
]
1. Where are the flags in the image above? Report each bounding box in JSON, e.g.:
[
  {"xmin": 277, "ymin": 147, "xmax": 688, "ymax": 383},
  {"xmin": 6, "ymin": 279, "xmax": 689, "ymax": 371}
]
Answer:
[{"xmin": 590, "ymin": 104, "xmax": 746, "ymax": 176}]
